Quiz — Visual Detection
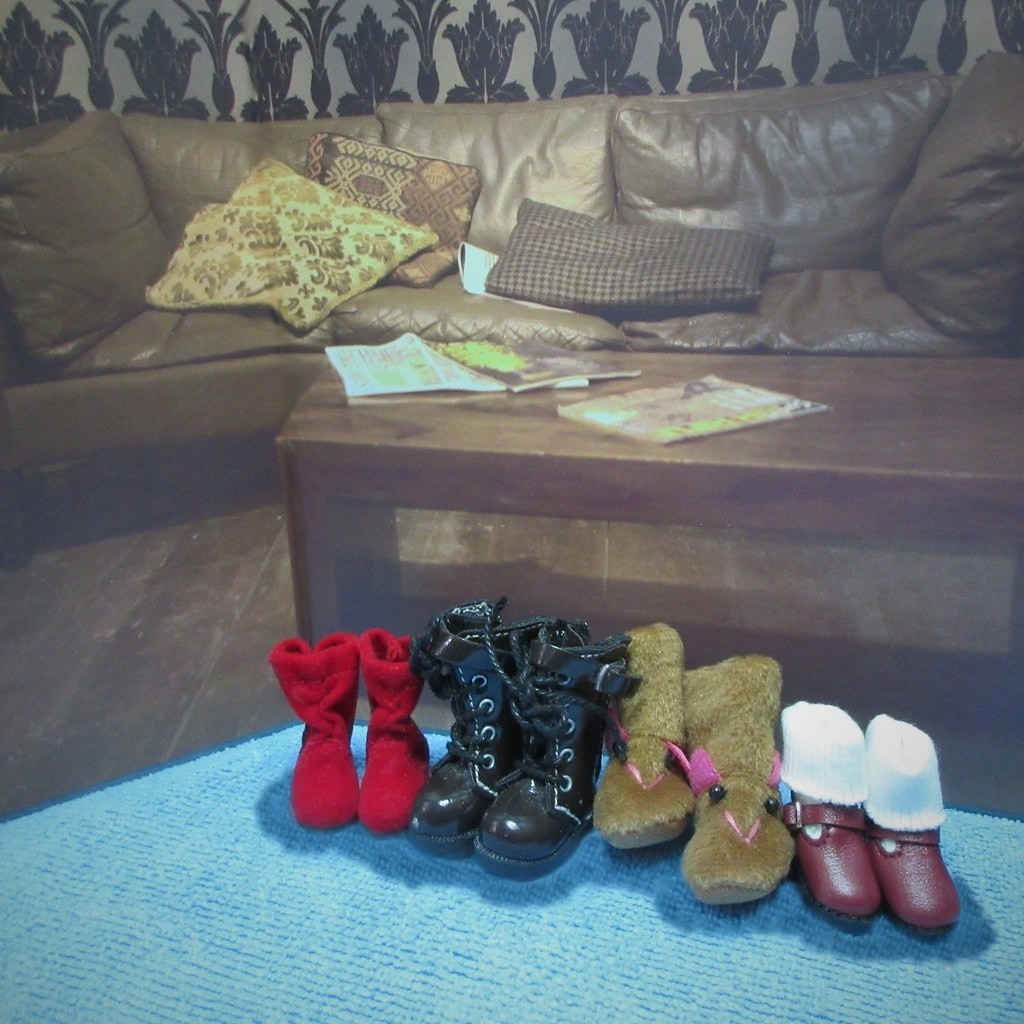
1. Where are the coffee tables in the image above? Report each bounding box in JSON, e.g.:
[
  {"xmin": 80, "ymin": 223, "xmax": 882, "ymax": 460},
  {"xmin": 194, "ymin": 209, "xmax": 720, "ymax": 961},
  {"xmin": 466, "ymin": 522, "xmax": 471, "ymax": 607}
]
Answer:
[{"xmin": 275, "ymin": 353, "xmax": 1024, "ymax": 727}]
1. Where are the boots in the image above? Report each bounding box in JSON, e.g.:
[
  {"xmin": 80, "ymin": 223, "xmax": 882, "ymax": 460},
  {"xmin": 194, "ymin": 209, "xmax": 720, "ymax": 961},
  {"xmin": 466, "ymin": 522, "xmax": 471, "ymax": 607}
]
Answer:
[
  {"xmin": 408, "ymin": 593, "xmax": 641, "ymax": 870},
  {"xmin": 775, "ymin": 704, "xmax": 961, "ymax": 932},
  {"xmin": 591, "ymin": 618, "xmax": 789, "ymax": 904},
  {"xmin": 270, "ymin": 627, "xmax": 431, "ymax": 833}
]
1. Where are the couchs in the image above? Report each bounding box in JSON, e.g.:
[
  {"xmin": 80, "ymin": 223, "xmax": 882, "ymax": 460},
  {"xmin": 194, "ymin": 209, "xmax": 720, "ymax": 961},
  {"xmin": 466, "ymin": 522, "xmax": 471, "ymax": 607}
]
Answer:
[{"xmin": 0, "ymin": 69, "xmax": 1024, "ymax": 571}]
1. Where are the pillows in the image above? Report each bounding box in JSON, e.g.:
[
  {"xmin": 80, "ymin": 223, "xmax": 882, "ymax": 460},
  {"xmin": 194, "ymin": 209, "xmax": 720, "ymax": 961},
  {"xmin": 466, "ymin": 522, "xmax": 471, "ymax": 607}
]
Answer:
[
  {"xmin": 145, "ymin": 158, "xmax": 439, "ymax": 334},
  {"xmin": 486, "ymin": 198, "xmax": 775, "ymax": 311},
  {"xmin": 878, "ymin": 52, "xmax": 1024, "ymax": 339},
  {"xmin": 304, "ymin": 132, "xmax": 482, "ymax": 286},
  {"xmin": 0, "ymin": 109, "xmax": 173, "ymax": 368}
]
